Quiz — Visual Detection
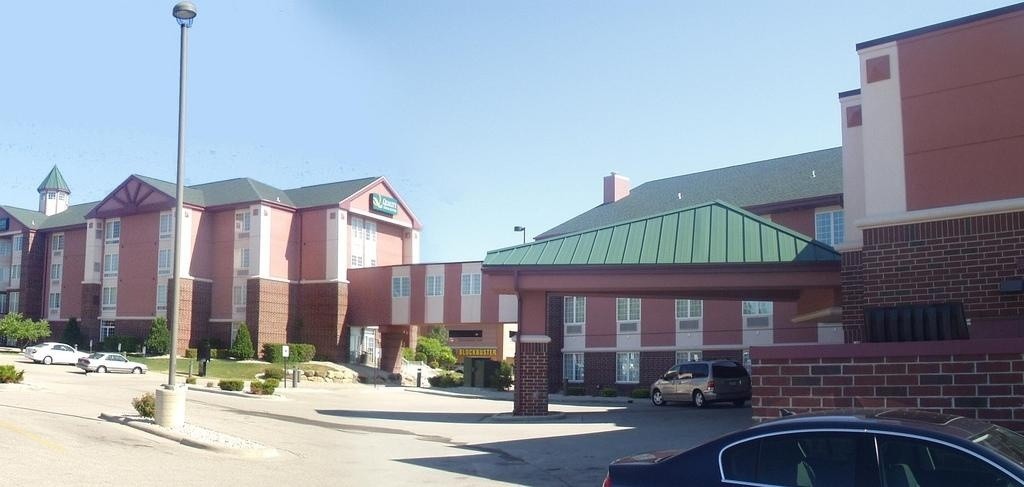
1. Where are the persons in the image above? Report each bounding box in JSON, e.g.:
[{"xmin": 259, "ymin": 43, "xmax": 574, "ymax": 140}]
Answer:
[{"xmin": 196, "ymin": 336, "xmax": 211, "ymax": 378}]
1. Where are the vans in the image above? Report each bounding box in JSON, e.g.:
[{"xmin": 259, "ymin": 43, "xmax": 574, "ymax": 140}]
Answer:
[{"xmin": 649, "ymin": 358, "xmax": 753, "ymax": 408}]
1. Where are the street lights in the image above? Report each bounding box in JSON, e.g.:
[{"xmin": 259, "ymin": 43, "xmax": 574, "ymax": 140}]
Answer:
[
  {"xmin": 156, "ymin": 0, "xmax": 196, "ymax": 428},
  {"xmin": 513, "ymin": 225, "xmax": 525, "ymax": 243}
]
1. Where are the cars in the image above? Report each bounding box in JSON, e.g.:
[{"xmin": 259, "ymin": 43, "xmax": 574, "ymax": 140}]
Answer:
[
  {"xmin": 23, "ymin": 341, "xmax": 87, "ymax": 365},
  {"xmin": 76, "ymin": 352, "xmax": 148, "ymax": 374},
  {"xmin": 603, "ymin": 406, "xmax": 1024, "ymax": 485}
]
